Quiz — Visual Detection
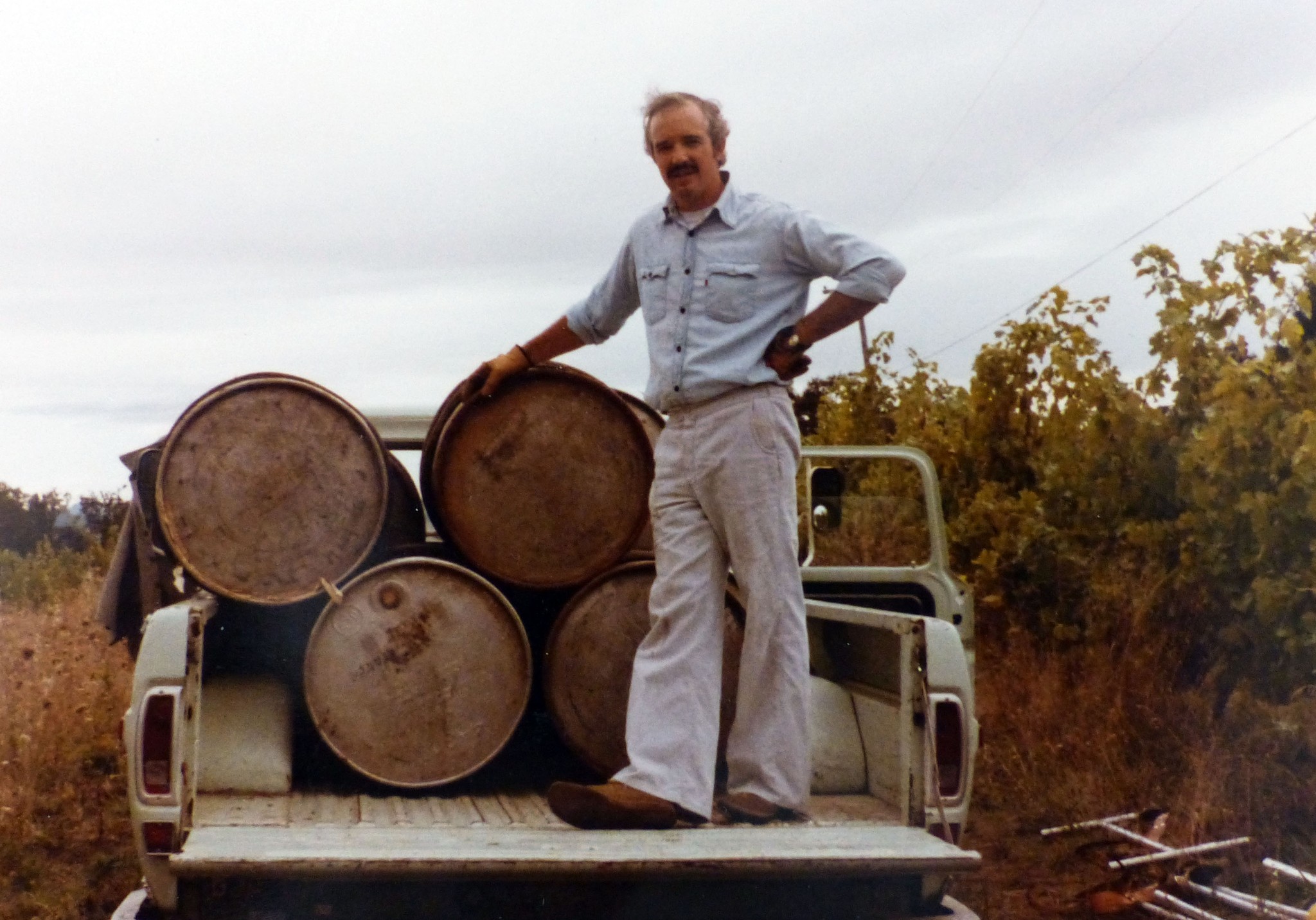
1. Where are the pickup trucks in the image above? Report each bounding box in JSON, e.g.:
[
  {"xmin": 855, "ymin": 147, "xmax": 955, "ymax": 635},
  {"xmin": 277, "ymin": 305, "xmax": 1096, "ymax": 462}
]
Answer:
[{"xmin": 104, "ymin": 406, "xmax": 985, "ymax": 920}]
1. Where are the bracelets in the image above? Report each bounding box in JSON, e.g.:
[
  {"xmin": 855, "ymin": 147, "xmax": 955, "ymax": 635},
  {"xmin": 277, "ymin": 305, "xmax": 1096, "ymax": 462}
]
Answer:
[{"xmin": 515, "ymin": 344, "xmax": 534, "ymax": 367}]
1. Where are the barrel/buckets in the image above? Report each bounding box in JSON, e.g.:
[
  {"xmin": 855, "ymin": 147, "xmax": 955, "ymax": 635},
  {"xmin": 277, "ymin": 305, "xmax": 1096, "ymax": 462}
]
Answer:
[
  {"xmin": 543, "ymin": 559, "xmax": 749, "ymax": 791},
  {"xmin": 386, "ymin": 450, "xmax": 426, "ymax": 557},
  {"xmin": 434, "ymin": 361, "xmax": 667, "ymax": 585},
  {"xmin": 304, "ymin": 557, "xmax": 533, "ymax": 788},
  {"xmin": 155, "ymin": 377, "xmax": 389, "ymax": 608}
]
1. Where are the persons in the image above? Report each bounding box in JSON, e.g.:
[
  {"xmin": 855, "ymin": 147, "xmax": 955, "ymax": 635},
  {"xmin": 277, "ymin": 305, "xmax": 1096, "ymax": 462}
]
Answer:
[{"xmin": 463, "ymin": 91, "xmax": 906, "ymax": 829}]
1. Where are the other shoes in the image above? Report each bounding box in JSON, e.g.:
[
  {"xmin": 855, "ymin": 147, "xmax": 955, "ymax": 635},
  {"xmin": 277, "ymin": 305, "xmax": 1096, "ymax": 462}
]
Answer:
[
  {"xmin": 718, "ymin": 792, "xmax": 782, "ymax": 821},
  {"xmin": 550, "ymin": 778, "xmax": 677, "ymax": 828}
]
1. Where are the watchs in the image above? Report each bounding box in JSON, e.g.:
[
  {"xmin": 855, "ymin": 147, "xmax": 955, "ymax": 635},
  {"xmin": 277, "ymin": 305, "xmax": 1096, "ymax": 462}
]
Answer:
[{"xmin": 776, "ymin": 324, "xmax": 813, "ymax": 353}]
